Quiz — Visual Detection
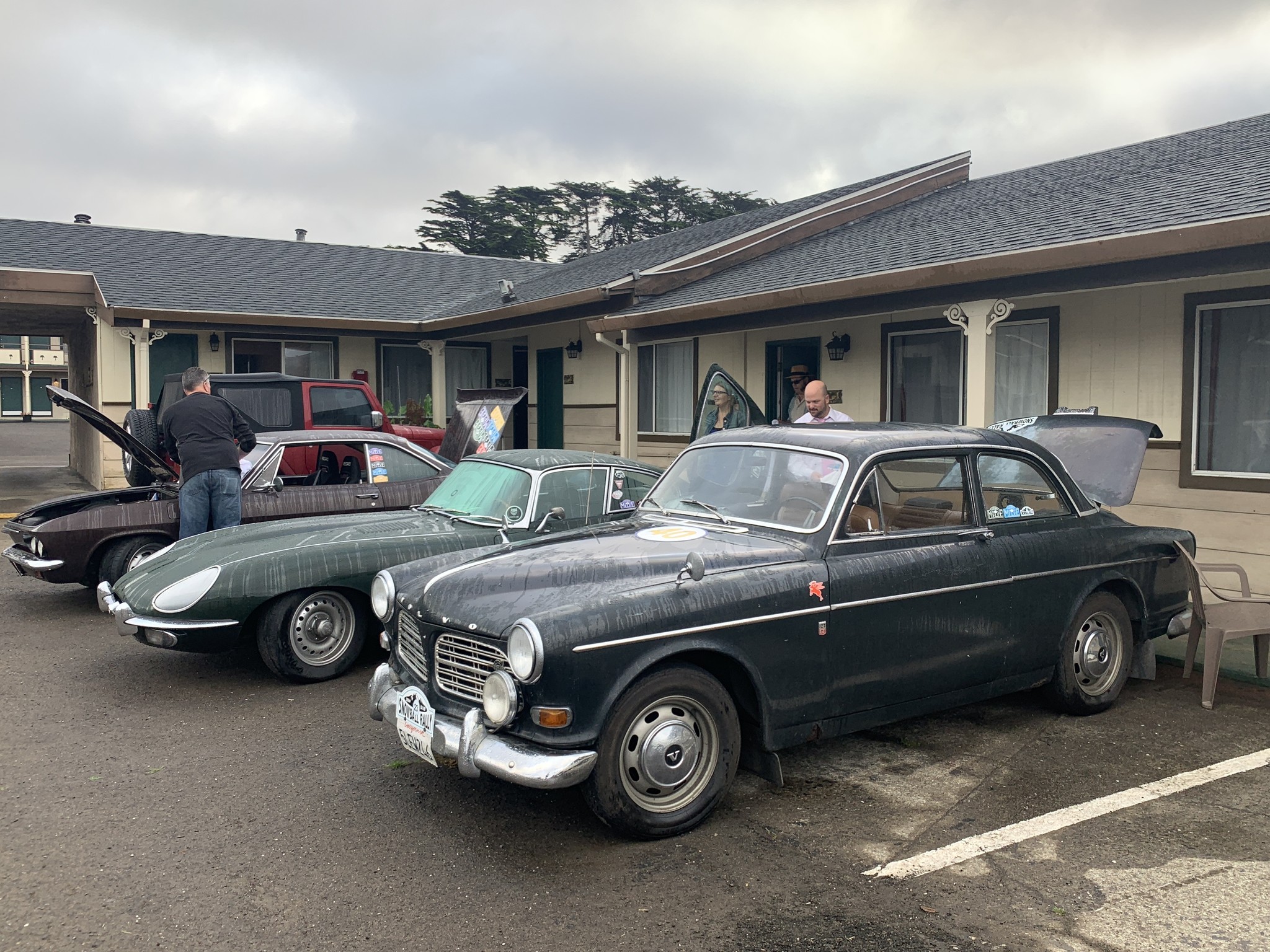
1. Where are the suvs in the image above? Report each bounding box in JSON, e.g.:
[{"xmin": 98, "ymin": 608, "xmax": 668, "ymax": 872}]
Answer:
[{"xmin": 121, "ymin": 370, "xmax": 447, "ymax": 486}]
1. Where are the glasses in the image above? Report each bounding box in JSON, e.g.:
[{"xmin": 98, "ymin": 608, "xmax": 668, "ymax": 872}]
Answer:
[
  {"xmin": 791, "ymin": 378, "xmax": 803, "ymax": 384},
  {"xmin": 710, "ymin": 390, "xmax": 728, "ymax": 395},
  {"xmin": 204, "ymin": 374, "xmax": 211, "ymax": 383}
]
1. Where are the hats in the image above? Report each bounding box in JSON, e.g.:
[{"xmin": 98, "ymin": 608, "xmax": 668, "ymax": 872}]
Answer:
[{"xmin": 784, "ymin": 365, "xmax": 813, "ymax": 379}]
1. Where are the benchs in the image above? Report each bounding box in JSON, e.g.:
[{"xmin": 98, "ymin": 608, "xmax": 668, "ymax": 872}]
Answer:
[{"xmin": 878, "ymin": 503, "xmax": 971, "ymax": 532}]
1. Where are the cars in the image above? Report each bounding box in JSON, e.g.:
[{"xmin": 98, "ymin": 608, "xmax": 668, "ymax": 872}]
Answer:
[
  {"xmin": 96, "ymin": 445, "xmax": 663, "ymax": 684},
  {"xmin": 0, "ymin": 385, "xmax": 458, "ymax": 588},
  {"xmin": 366, "ymin": 402, "xmax": 1197, "ymax": 844}
]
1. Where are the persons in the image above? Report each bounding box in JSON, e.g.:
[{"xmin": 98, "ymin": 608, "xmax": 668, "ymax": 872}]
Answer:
[
  {"xmin": 161, "ymin": 366, "xmax": 257, "ymax": 540},
  {"xmin": 703, "ymin": 382, "xmax": 746, "ymax": 435},
  {"xmin": 787, "ymin": 380, "xmax": 854, "ymax": 486},
  {"xmin": 779, "ymin": 366, "xmax": 815, "ymax": 423}
]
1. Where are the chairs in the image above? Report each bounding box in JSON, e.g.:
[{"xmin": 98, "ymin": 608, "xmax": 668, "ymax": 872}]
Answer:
[
  {"xmin": 1172, "ymin": 538, "xmax": 1270, "ymax": 709},
  {"xmin": 333, "ymin": 456, "xmax": 361, "ymax": 482},
  {"xmin": 816, "ymin": 499, "xmax": 885, "ymax": 534},
  {"xmin": 302, "ymin": 450, "xmax": 339, "ymax": 484},
  {"xmin": 775, "ymin": 482, "xmax": 829, "ymax": 528}
]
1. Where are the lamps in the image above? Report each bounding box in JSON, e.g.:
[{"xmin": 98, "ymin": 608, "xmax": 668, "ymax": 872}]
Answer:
[
  {"xmin": 54, "ymin": 378, "xmax": 59, "ymax": 384},
  {"xmin": 563, "ymin": 338, "xmax": 582, "ymax": 359},
  {"xmin": 208, "ymin": 332, "xmax": 221, "ymax": 351},
  {"xmin": 824, "ymin": 331, "xmax": 851, "ymax": 362},
  {"xmin": 497, "ymin": 277, "xmax": 515, "ymax": 301}
]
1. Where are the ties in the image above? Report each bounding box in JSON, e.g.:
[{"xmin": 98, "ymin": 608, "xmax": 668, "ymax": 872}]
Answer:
[{"xmin": 814, "ymin": 418, "xmax": 835, "ymax": 477}]
